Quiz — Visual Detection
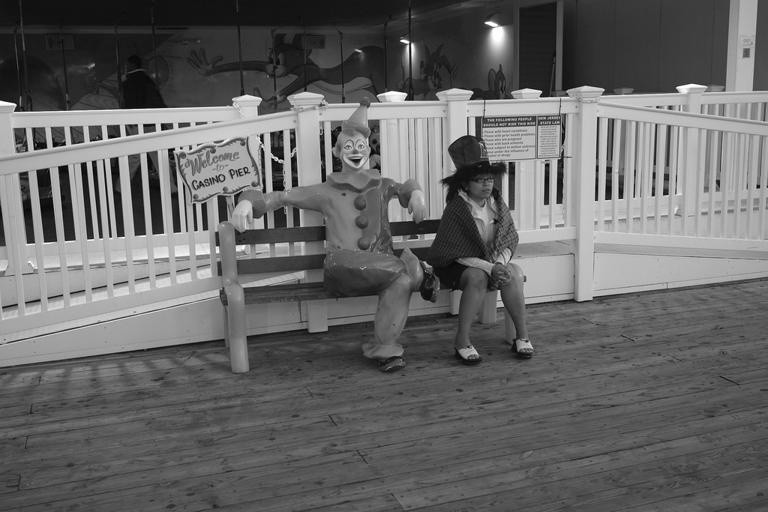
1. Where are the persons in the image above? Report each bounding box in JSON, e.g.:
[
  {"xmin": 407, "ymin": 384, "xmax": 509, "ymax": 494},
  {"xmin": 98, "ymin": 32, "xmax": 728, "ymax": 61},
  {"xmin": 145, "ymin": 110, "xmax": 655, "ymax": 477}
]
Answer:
[
  {"xmin": 235, "ymin": 97, "xmax": 440, "ymax": 373},
  {"xmin": 111, "ymin": 55, "xmax": 177, "ymax": 200},
  {"xmin": 427, "ymin": 135, "xmax": 534, "ymax": 364}
]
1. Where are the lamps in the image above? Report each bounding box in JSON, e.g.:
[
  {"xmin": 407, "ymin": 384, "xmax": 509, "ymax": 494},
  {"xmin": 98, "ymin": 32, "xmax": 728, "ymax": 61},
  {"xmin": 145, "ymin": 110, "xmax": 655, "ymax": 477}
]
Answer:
[
  {"xmin": 484, "ymin": 14, "xmax": 501, "ymax": 28},
  {"xmin": 399, "ymin": 33, "xmax": 410, "ymax": 45}
]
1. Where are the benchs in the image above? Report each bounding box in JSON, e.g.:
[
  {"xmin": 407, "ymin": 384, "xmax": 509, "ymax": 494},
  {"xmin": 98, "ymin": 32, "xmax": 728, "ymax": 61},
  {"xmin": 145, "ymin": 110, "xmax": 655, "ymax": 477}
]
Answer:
[{"xmin": 215, "ymin": 221, "xmax": 516, "ymax": 375}]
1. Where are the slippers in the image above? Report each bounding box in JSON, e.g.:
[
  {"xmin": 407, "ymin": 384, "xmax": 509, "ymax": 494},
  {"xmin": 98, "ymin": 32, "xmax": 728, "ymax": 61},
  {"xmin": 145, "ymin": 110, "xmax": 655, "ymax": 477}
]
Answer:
[
  {"xmin": 512, "ymin": 339, "xmax": 534, "ymax": 359},
  {"xmin": 455, "ymin": 345, "xmax": 482, "ymax": 365}
]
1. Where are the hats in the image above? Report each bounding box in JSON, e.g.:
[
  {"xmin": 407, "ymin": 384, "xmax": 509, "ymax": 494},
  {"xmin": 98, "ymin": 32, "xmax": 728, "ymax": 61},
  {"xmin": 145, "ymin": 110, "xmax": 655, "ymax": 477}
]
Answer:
[{"xmin": 448, "ymin": 136, "xmax": 489, "ymax": 169}]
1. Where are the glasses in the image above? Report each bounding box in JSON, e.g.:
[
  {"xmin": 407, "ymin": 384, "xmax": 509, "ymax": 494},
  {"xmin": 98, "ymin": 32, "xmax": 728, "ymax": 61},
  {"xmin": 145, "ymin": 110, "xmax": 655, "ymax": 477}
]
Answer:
[{"xmin": 470, "ymin": 178, "xmax": 496, "ymax": 183}]
